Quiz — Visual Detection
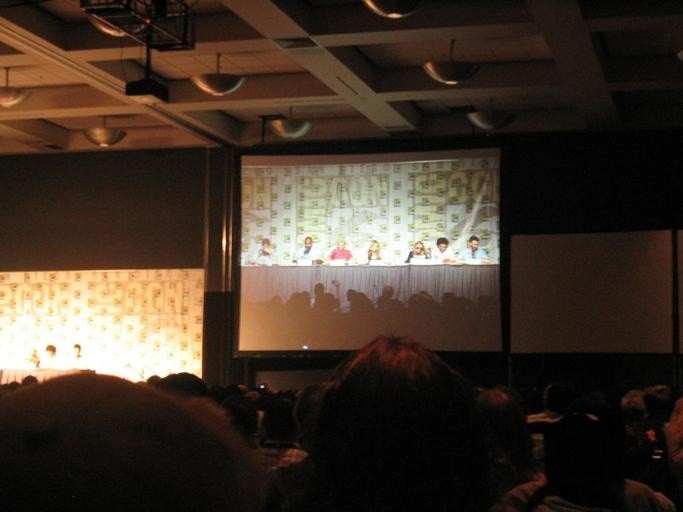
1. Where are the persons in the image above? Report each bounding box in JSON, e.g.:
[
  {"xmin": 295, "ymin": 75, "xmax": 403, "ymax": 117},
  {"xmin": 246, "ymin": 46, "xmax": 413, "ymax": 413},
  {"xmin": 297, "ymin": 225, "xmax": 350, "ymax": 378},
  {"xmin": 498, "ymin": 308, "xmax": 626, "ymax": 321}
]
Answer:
[
  {"xmin": 47, "ymin": 343, "xmax": 85, "ymax": 369},
  {"xmin": 428, "ymin": 237, "xmax": 456, "ymax": 263},
  {"xmin": 237, "ymin": 279, "xmax": 501, "ymax": 352},
  {"xmin": 403, "ymin": 240, "xmax": 430, "ymax": 264},
  {"xmin": 458, "ymin": 234, "xmax": 492, "ymax": 263},
  {"xmin": 327, "ymin": 240, "xmax": 356, "ymax": 264},
  {"xmin": 276, "ymin": 338, "xmax": 474, "ymax": 510},
  {"xmin": 157, "ymin": 374, "xmax": 260, "ymax": 445},
  {"xmin": 290, "ymin": 236, "xmax": 325, "ymax": 266},
  {"xmin": 1, "ymin": 374, "xmax": 262, "ymax": 509},
  {"xmin": 247, "ymin": 238, "xmax": 278, "ymax": 266},
  {"xmin": 237, "ymin": 384, "xmax": 334, "ymax": 470},
  {"xmin": 2, "ymin": 377, "xmax": 38, "ymax": 392},
  {"xmin": 363, "ymin": 239, "xmax": 383, "ymax": 265},
  {"xmin": 480, "ymin": 385, "xmax": 682, "ymax": 510}
]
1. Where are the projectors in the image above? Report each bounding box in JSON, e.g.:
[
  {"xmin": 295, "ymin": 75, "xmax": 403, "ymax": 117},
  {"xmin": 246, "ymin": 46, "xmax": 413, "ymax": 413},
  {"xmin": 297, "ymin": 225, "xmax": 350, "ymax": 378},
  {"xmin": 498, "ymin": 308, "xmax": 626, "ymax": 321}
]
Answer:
[{"xmin": 125, "ymin": 79, "xmax": 169, "ymax": 105}]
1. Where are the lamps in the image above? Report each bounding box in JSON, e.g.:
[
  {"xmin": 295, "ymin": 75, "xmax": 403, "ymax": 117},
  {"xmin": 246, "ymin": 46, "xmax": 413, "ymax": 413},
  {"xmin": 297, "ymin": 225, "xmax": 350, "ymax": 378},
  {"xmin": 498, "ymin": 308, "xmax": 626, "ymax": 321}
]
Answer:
[
  {"xmin": 87, "ymin": 8, "xmax": 148, "ymax": 38},
  {"xmin": 421, "ymin": 39, "xmax": 479, "ymax": 84},
  {"xmin": 81, "ymin": 116, "xmax": 128, "ymax": 147},
  {"xmin": 191, "ymin": 52, "xmax": 244, "ymax": 95},
  {"xmin": 0, "ymin": 66, "xmax": 31, "ymax": 107},
  {"xmin": 468, "ymin": 96, "xmax": 513, "ymax": 130},
  {"xmin": 269, "ymin": 105, "xmax": 312, "ymax": 138},
  {"xmin": 365, "ymin": 0, "xmax": 422, "ymax": 18}
]
